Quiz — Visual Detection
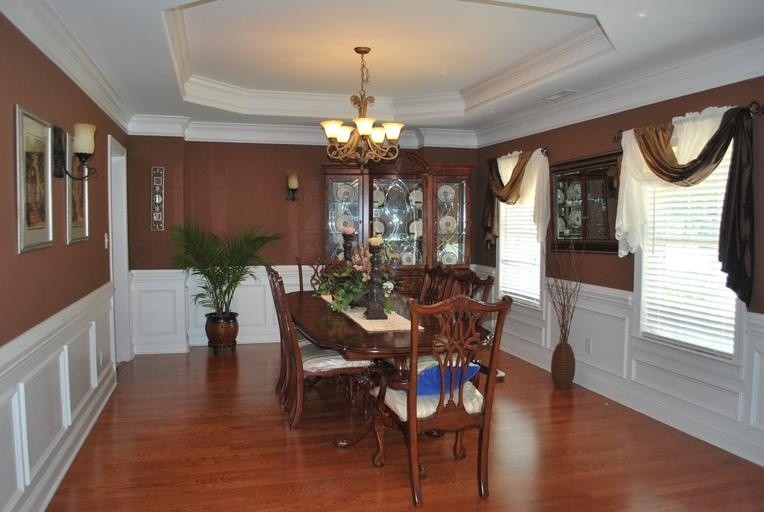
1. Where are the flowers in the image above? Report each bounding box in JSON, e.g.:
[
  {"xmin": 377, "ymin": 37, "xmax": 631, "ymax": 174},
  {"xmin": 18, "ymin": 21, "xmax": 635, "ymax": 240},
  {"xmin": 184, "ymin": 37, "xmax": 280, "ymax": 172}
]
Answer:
[{"xmin": 320, "ymin": 242, "xmax": 404, "ymax": 313}]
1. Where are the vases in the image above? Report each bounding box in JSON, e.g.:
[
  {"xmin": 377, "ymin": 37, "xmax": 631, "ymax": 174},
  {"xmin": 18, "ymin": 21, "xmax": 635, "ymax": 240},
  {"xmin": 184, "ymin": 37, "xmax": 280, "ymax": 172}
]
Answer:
[{"xmin": 551, "ymin": 342, "xmax": 577, "ymax": 390}]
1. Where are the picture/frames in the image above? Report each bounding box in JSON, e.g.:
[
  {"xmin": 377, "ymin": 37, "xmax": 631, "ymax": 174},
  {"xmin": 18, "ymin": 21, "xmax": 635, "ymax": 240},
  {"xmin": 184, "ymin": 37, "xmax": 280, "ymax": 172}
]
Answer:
[
  {"xmin": 13, "ymin": 104, "xmax": 55, "ymax": 257},
  {"xmin": 65, "ymin": 131, "xmax": 92, "ymax": 246},
  {"xmin": 549, "ymin": 147, "xmax": 625, "ymax": 255}
]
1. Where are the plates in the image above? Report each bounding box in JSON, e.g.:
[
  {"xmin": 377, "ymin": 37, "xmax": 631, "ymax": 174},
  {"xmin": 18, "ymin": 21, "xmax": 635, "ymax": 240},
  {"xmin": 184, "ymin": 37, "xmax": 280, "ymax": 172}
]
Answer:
[
  {"xmin": 401, "ymin": 185, "xmax": 458, "ymax": 266},
  {"xmin": 336, "ymin": 184, "xmax": 386, "ymax": 237},
  {"xmin": 568, "ymin": 181, "xmax": 584, "ymax": 229}
]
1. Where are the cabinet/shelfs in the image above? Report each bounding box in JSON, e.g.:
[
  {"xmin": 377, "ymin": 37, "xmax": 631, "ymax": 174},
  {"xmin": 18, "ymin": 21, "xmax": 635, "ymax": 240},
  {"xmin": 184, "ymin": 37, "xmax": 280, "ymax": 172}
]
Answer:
[{"xmin": 320, "ymin": 161, "xmax": 476, "ymax": 297}]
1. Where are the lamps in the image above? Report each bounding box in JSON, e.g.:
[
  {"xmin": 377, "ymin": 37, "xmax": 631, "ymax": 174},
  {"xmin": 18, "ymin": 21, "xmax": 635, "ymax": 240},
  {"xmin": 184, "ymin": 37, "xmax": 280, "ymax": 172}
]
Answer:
[
  {"xmin": 54, "ymin": 121, "xmax": 96, "ymax": 181},
  {"xmin": 286, "ymin": 173, "xmax": 301, "ymax": 202},
  {"xmin": 319, "ymin": 46, "xmax": 406, "ymax": 173}
]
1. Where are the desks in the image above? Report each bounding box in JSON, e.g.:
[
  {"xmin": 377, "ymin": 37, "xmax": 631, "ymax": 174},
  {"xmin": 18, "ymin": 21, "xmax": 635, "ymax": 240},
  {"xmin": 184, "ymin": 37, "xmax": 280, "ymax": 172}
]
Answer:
[{"xmin": 284, "ymin": 289, "xmax": 492, "ymax": 469}]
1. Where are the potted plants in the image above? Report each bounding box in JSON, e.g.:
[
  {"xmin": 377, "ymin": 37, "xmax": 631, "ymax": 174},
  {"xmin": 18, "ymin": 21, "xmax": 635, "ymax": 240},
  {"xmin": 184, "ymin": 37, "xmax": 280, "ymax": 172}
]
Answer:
[{"xmin": 169, "ymin": 218, "xmax": 287, "ymax": 355}]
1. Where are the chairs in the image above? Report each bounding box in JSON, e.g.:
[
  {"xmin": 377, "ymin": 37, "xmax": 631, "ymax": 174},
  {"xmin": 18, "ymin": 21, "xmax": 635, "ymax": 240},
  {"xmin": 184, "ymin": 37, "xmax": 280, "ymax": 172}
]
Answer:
[
  {"xmin": 392, "ymin": 262, "xmax": 498, "ymax": 374},
  {"xmin": 369, "ymin": 294, "xmax": 513, "ymax": 507},
  {"xmin": 265, "ymin": 264, "xmax": 374, "ymax": 431}
]
